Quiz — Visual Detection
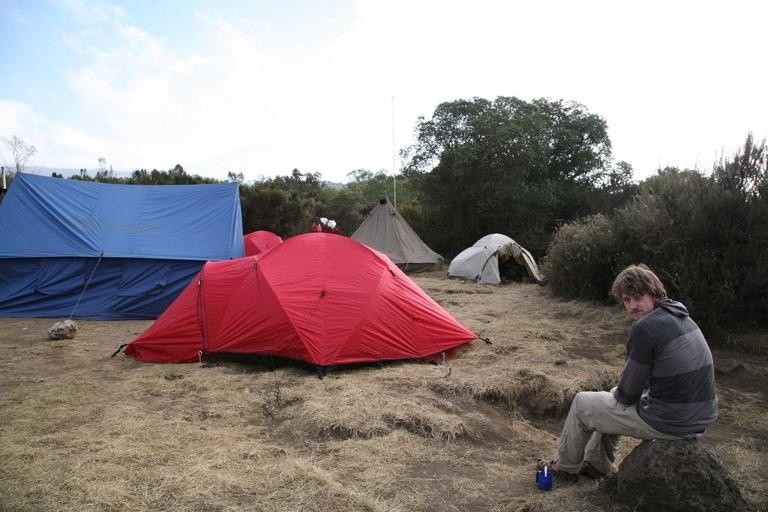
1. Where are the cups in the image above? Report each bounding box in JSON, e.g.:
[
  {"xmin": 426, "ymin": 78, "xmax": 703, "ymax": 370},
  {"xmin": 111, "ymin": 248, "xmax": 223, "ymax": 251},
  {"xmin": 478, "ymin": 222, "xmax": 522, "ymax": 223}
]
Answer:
[{"xmin": 537, "ymin": 463, "xmax": 553, "ymax": 491}]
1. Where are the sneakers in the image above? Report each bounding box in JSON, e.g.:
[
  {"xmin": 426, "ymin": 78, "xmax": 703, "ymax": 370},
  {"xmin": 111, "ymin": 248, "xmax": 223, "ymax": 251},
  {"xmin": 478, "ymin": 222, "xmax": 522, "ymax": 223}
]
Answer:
[
  {"xmin": 577, "ymin": 460, "xmax": 607, "ymax": 482},
  {"xmin": 535, "ymin": 457, "xmax": 578, "ymax": 483}
]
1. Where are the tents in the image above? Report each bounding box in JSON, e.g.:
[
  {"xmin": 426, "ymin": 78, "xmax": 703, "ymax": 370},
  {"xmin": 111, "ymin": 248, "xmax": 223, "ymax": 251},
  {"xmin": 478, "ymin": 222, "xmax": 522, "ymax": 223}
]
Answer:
[
  {"xmin": 348, "ymin": 194, "xmax": 447, "ymax": 275},
  {"xmin": 241, "ymin": 229, "xmax": 284, "ymax": 258},
  {"xmin": 447, "ymin": 233, "xmax": 541, "ymax": 286},
  {"xmin": 122, "ymin": 232, "xmax": 477, "ymax": 368},
  {"xmin": 0, "ymin": 173, "xmax": 245, "ymax": 321}
]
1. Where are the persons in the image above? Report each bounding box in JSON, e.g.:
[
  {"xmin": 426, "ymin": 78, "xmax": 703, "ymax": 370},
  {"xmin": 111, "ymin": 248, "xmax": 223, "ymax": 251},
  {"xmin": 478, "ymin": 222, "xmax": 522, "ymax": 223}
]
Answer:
[{"xmin": 537, "ymin": 263, "xmax": 721, "ymax": 483}]
